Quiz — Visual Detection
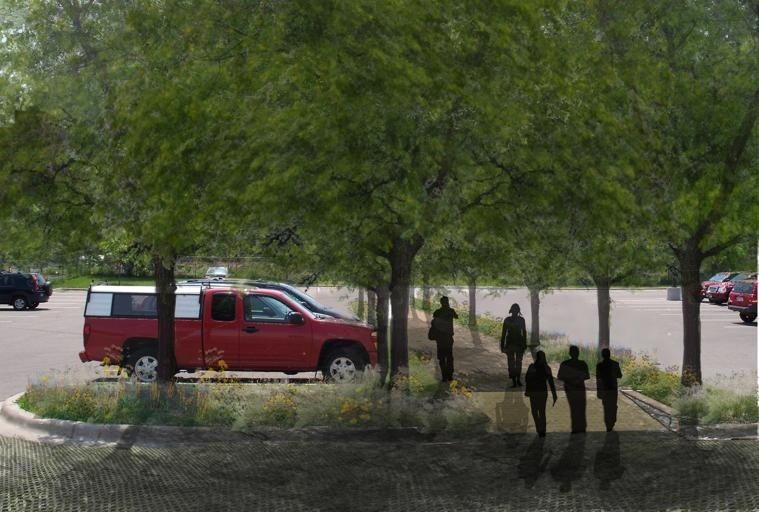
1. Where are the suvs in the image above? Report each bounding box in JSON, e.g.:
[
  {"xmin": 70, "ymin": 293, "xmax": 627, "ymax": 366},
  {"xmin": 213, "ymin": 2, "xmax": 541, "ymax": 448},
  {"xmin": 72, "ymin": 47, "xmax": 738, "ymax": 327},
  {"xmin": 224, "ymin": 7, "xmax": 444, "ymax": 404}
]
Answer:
[{"xmin": 0, "ymin": 270, "xmax": 53, "ymax": 312}]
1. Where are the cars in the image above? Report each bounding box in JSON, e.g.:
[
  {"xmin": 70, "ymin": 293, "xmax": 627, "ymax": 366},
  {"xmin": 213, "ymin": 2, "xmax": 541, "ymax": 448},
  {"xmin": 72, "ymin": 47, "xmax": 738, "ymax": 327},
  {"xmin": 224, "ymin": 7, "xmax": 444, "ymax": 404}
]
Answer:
[
  {"xmin": 705, "ymin": 271, "xmax": 758, "ymax": 303},
  {"xmin": 698, "ymin": 270, "xmax": 739, "ymax": 303},
  {"xmin": 726, "ymin": 276, "xmax": 757, "ymax": 323}
]
